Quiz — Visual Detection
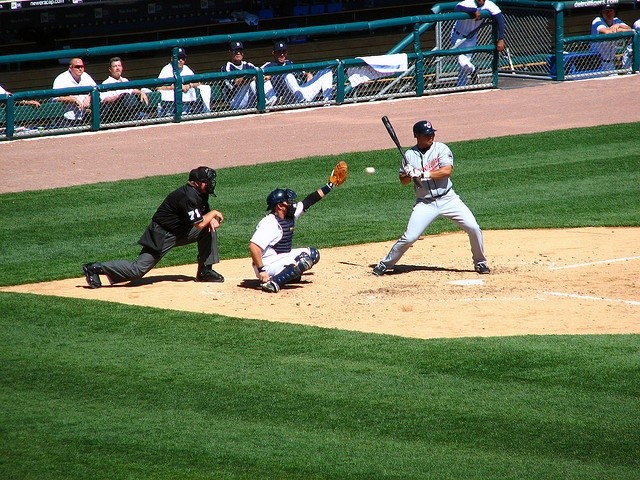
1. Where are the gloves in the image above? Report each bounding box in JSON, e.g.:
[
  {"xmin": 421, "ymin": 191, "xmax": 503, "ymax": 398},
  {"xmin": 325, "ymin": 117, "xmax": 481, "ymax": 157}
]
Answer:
[
  {"xmin": 404, "ymin": 163, "xmax": 414, "ymax": 177},
  {"xmin": 409, "ymin": 169, "xmax": 424, "ymax": 179}
]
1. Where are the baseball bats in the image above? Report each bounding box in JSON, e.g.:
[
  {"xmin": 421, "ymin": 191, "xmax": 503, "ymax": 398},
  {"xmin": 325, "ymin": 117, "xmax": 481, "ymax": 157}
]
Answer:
[{"xmin": 382, "ymin": 116, "xmax": 423, "ymax": 188}]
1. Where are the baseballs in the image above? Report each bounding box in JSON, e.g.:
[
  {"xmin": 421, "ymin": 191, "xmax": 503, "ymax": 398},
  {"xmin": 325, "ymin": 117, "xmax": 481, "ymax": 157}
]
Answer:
[{"xmin": 366, "ymin": 167, "xmax": 376, "ymax": 174}]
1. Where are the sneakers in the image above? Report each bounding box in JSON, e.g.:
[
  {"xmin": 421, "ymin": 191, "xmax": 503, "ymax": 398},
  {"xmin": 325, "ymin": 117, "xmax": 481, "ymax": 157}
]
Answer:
[
  {"xmin": 324, "ymin": 87, "xmax": 333, "ymax": 107},
  {"xmin": 181, "ymin": 111, "xmax": 188, "ymax": 115},
  {"xmin": 138, "ymin": 111, "xmax": 151, "ymax": 119},
  {"xmin": 256, "ymin": 281, "xmax": 280, "ymax": 293},
  {"xmin": 472, "ymin": 66, "xmax": 480, "ymax": 85},
  {"xmin": 294, "ymin": 90, "xmax": 304, "ymax": 103},
  {"xmin": 165, "ymin": 113, "xmax": 171, "ymax": 117},
  {"xmin": 264, "ymin": 95, "xmax": 278, "ymax": 112},
  {"xmin": 255, "ymin": 98, "xmax": 272, "ymax": 108},
  {"xmin": 372, "ymin": 261, "xmax": 387, "ymax": 276},
  {"xmin": 474, "ymin": 262, "xmax": 490, "ymax": 274},
  {"xmin": 83, "ymin": 263, "xmax": 101, "ymax": 288},
  {"xmin": 194, "ymin": 270, "xmax": 224, "ymax": 282}
]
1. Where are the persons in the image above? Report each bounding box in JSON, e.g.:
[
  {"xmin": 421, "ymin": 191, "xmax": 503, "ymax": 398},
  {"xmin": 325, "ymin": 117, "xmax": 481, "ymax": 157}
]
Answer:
[
  {"xmin": 96, "ymin": 58, "xmax": 151, "ymax": 106},
  {"xmin": 248, "ymin": 160, "xmax": 349, "ymax": 293},
  {"xmin": 52, "ymin": 57, "xmax": 98, "ymax": 110},
  {"xmin": 0, "ymin": 86, "xmax": 40, "ymax": 109},
  {"xmin": 155, "ymin": 49, "xmax": 211, "ymax": 118},
  {"xmin": 448, "ymin": 0, "xmax": 505, "ymax": 86},
  {"xmin": 371, "ymin": 115, "xmax": 492, "ymax": 277},
  {"xmin": 81, "ymin": 166, "xmax": 225, "ymax": 289},
  {"xmin": 220, "ymin": 41, "xmax": 277, "ymax": 109},
  {"xmin": 590, "ymin": 6, "xmax": 633, "ymax": 76},
  {"xmin": 257, "ymin": 44, "xmax": 335, "ymax": 107}
]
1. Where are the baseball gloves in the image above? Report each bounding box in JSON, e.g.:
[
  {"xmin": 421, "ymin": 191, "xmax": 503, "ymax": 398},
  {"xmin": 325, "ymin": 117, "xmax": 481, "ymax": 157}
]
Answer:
[{"xmin": 330, "ymin": 161, "xmax": 347, "ymax": 185}]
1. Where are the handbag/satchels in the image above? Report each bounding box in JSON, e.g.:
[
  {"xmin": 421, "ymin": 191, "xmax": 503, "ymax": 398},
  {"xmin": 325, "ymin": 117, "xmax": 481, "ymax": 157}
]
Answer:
[{"xmin": 137, "ymin": 220, "xmax": 170, "ymax": 256}]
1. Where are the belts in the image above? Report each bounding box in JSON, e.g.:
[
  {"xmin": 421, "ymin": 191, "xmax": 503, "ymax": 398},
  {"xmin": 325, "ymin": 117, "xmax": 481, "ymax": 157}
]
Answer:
[
  {"xmin": 454, "ymin": 28, "xmax": 475, "ymax": 39},
  {"xmin": 601, "ymin": 58, "xmax": 614, "ymax": 63}
]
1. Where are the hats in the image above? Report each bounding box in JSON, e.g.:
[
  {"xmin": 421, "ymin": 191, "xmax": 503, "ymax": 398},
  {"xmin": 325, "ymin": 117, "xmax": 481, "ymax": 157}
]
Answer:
[
  {"xmin": 170, "ymin": 47, "xmax": 187, "ymax": 57},
  {"xmin": 229, "ymin": 41, "xmax": 244, "ymax": 50},
  {"xmin": 602, "ymin": 4, "xmax": 615, "ymax": 10},
  {"xmin": 273, "ymin": 41, "xmax": 287, "ymax": 51}
]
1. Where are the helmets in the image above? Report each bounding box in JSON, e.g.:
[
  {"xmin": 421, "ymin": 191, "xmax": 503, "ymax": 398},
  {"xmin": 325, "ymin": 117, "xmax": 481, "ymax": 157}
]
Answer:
[
  {"xmin": 189, "ymin": 166, "xmax": 218, "ymax": 197},
  {"xmin": 413, "ymin": 120, "xmax": 437, "ymax": 136},
  {"xmin": 266, "ymin": 188, "xmax": 297, "ymax": 218}
]
